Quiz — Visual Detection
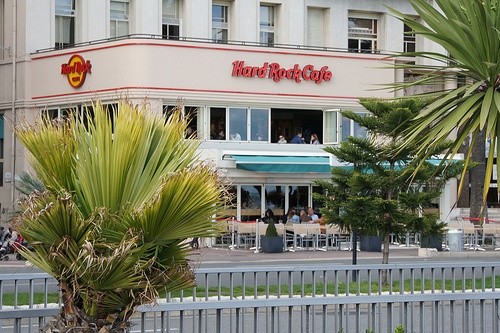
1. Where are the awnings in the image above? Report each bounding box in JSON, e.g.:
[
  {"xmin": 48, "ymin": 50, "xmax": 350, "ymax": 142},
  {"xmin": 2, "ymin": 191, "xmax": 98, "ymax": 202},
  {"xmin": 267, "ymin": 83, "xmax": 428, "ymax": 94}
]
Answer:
[{"xmin": 232, "ymin": 156, "xmax": 458, "ymax": 173}]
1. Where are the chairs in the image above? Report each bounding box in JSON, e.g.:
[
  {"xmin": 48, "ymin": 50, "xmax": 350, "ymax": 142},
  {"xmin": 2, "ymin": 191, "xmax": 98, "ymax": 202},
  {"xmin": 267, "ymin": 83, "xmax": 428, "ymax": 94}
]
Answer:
[
  {"xmin": 441, "ymin": 221, "xmax": 500, "ymax": 252},
  {"xmin": 217, "ymin": 221, "xmax": 349, "ymax": 251}
]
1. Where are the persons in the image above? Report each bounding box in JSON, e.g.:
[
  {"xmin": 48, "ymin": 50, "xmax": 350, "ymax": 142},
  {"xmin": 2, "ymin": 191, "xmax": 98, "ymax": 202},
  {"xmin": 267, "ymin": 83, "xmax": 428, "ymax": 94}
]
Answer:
[
  {"xmin": 289, "ymin": 131, "xmax": 304, "ymax": 144},
  {"xmin": 278, "ymin": 135, "xmax": 287, "ymax": 144},
  {"xmin": 282, "ymin": 208, "xmax": 339, "ymax": 247},
  {"xmin": 185, "ymin": 121, "xmax": 241, "ymax": 141},
  {"xmin": 0, "ymin": 227, "xmax": 24, "ymax": 256},
  {"xmin": 310, "ymin": 134, "xmax": 320, "ymax": 144},
  {"xmin": 261, "ymin": 209, "xmax": 279, "ymax": 224}
]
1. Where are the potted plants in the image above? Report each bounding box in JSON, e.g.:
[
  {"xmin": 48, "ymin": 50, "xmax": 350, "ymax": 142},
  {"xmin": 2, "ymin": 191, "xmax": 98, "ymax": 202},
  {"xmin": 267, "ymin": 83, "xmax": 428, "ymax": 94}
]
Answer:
[
  {"xmin": 423, "ymin": 221, "xmax": 443, "ymax": 250},
  {"xmin": 259, "ymin": 220, "xmax": 284, "ymax": 255},
  {"xmin": 360, "ymin": 229, "xmax": 381, "ymax": 252}
]
